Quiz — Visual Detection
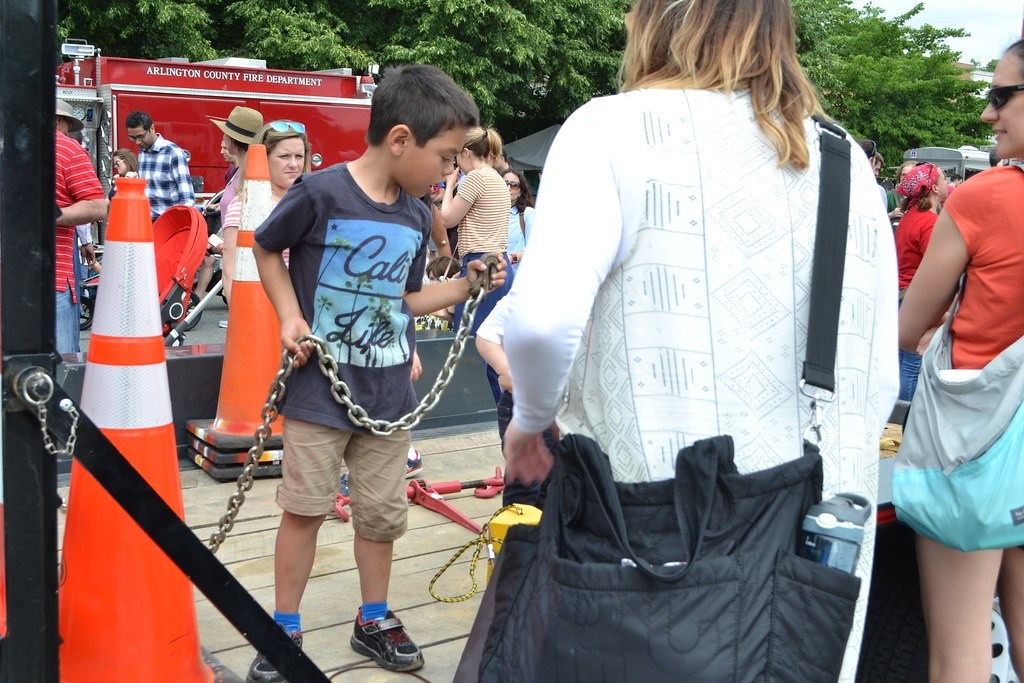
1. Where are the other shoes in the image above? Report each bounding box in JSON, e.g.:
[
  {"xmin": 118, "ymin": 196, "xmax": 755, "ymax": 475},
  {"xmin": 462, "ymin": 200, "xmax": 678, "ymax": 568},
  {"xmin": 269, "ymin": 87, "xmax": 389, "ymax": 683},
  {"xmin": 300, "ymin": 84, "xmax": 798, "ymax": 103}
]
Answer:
[
  {"xmin": 219, "ymin": 320, "xmax": 228, "ymax": 328},
  {"xmin": 405, "ymin": 448, "xmax": 424, "ymax": 479}
]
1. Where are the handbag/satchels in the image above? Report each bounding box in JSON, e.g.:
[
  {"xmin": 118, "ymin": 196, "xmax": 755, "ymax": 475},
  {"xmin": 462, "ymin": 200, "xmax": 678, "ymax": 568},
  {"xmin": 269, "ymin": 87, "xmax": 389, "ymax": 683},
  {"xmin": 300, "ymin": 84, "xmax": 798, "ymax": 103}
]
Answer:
[
  {"xmin": 476, "ymin": 433, "xmax": 862, "ymax": 683},
  {"xmin": 890, "ymin": 324, "xmax": 1024, "ymax": 552}
]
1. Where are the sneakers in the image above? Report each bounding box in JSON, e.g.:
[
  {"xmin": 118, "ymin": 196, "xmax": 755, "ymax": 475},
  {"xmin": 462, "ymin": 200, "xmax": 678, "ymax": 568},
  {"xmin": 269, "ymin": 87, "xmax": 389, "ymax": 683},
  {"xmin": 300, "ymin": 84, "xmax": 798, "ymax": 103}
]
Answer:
[
  {"xmin": 350, "ymin": 606, "xmax": 425, "ymax": 673},
  {"xmin": 245, "ymin": 631, "xmax": 303, "ymax": 683}
]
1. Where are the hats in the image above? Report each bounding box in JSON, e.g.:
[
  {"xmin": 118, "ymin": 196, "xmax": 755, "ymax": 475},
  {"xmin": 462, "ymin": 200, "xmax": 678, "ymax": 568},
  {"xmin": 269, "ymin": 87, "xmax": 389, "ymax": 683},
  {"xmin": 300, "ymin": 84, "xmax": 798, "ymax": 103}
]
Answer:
[
  {"xmin": 55, "ymin": 98, "xmax": 85, "ymax": 132},
  {"xmin": 209, "ymin": 106, "xmax": 264, "ymax": 145}
]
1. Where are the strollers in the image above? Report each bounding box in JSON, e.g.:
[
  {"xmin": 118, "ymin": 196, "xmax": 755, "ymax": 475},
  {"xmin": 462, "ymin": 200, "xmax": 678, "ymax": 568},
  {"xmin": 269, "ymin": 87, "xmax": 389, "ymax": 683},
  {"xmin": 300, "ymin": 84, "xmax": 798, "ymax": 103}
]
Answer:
[{"xmin": 79, "ymin": 190, "xmax": 223, "ymax": 348}]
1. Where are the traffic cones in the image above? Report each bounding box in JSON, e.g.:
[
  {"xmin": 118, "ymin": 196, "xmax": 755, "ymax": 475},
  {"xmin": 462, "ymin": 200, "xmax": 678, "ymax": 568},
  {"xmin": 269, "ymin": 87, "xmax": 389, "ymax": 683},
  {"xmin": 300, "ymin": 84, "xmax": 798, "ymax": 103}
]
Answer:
[{"xmin": 60, "ymin": 172, "xmax": 217, "ymax": 683}]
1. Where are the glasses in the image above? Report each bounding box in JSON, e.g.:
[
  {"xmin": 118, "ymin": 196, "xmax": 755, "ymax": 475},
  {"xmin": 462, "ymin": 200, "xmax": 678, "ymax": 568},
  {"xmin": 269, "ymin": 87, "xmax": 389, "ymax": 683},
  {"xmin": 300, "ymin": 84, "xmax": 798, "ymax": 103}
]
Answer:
[
  {"xmin": 60, "ymin": 116, "xmax": 73, "ymax": 130},
  {"xmin": 269, "ymin": 122, "xmax": 306, "ymax": 134},
  {"xmin": 916, "ymin": 161, "xmax": 933, "ymax": 187},
  {"xmin": 988, "ymin": 85, "xmax": 1024, "ymax": 110},
  {"xmin": 505, "ymin": 180, "xmax": 521, "ymax": 190},
  {"xmin": 114, "ymin": 158, "xmax": 128, "ymax": 166},
  {"xmin": 127, "ymin": 129, "xmax": 150, "ymax": 141}
]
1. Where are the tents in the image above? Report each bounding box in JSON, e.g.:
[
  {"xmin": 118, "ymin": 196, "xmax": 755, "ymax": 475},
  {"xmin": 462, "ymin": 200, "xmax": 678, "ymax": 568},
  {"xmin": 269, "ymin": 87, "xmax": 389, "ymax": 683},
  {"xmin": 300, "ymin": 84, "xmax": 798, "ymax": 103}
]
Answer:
[{"xmin": 501, "ymin": 123, "xmax": 562, "ymax": 206}]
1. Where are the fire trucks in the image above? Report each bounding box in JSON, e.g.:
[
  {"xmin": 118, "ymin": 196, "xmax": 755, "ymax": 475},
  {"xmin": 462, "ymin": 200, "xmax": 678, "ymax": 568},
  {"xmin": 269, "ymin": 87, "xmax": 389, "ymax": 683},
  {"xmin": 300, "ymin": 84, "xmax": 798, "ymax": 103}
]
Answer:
[{"xmin": 56, "ymin": 38, "xmax": 377, "ymax": 256}]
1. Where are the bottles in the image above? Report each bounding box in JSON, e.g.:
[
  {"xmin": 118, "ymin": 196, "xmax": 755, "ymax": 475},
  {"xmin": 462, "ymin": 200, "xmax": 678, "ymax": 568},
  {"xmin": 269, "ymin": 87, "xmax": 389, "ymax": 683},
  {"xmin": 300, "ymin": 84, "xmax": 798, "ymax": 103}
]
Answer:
[{"xmin": 794, "ymin": 493, "xmax": 871, "ymax": 577}]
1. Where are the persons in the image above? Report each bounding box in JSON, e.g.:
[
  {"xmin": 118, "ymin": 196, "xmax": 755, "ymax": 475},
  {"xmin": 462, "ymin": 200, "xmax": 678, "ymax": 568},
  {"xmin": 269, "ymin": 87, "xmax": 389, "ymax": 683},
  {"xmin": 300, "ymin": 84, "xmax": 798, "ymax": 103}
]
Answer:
[
  {"xmin": 503, "ymin": 0, "xmax": 900, "ymax": 683},
  {"xmin": 857, "ymin": 138, "xmax": 1015, "ymax": 402},
  {"xmin": 246, "ymin": 63, "xmax": 510, "ymax": 683},
  {"xmin": 899, "ymin": 44, "xmax": 1024, "ymax": 683},
  {"xmin": 56, "ymin": 95, "xmax": 543, "ymax": 479}
]
